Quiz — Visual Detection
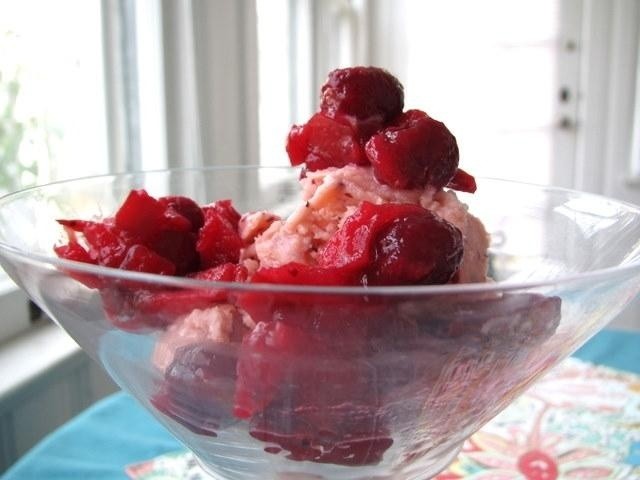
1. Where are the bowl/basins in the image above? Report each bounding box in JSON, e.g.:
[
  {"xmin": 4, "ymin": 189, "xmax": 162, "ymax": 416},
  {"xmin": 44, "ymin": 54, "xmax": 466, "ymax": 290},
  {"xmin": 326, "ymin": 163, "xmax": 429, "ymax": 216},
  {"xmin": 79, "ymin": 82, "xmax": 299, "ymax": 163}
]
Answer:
[{"xmin": 0, "ymin": 164, "xmax": 640, "ymax": 479}]
